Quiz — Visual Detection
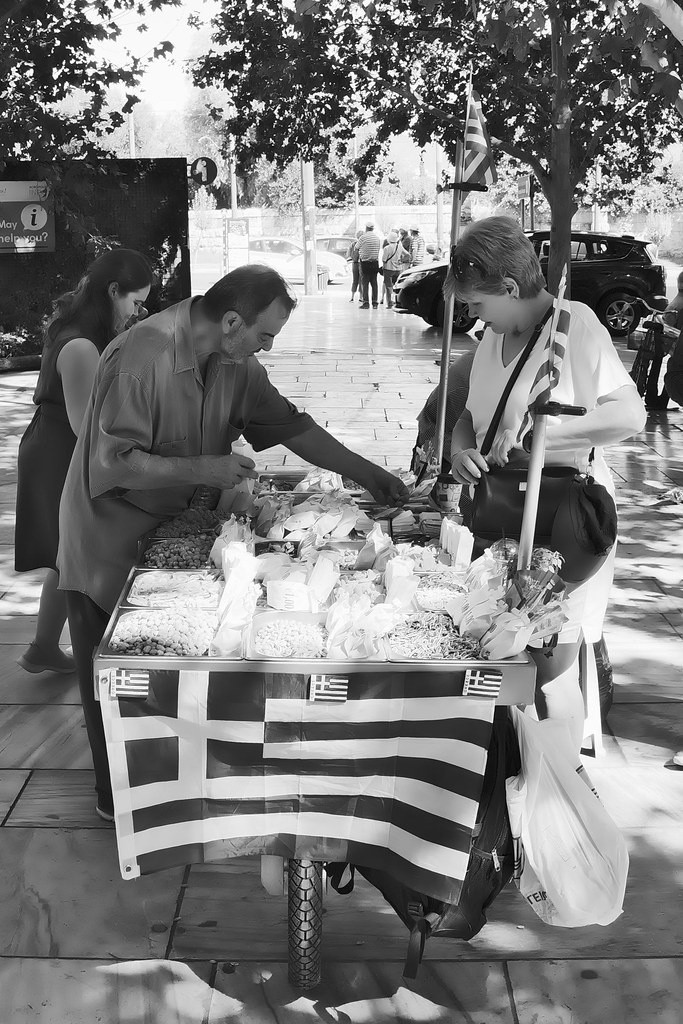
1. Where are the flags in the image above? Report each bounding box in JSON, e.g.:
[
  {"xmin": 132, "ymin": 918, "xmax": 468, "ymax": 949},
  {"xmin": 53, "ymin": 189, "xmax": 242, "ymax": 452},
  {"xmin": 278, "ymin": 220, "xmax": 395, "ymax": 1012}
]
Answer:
[{"xmin": 461, "ymin": 90, "xmax": 499, "ymax": 205}]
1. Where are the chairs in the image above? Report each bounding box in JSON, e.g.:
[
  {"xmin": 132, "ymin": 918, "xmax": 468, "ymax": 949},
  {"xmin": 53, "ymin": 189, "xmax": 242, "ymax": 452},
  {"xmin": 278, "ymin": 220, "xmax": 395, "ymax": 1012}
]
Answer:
[{"xmin": 540, "ymin": 244, "xmax": 550, "ymax": 263}]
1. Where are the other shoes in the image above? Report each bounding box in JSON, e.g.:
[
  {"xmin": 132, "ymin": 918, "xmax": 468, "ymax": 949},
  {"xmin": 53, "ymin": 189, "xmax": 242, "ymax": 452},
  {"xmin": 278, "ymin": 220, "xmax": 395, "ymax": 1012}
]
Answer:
[
  {"xmin": 387, "ymin": 305, "xmax": 392, "ymax": 309},
  {"xmin": 373, "ymin": 305, "xmax": 378, "ymax": 309},
  {"xmin": 359, "ymin": 299, "xmax": 364, "ymax": 302},
  {"xmin": 95, "ymin": 805, "xmax": 116, "ymax": 822},
  {"xmin": 359, "ymin": 305, "xmax": 369, "ymax": 309},
  {"xmin": 673, "ymin": 750, "xmax": 683, "ymax": 766},
  {"xmin": 349, "ymin": 299, "xmax": 353, "ymax": 302}
]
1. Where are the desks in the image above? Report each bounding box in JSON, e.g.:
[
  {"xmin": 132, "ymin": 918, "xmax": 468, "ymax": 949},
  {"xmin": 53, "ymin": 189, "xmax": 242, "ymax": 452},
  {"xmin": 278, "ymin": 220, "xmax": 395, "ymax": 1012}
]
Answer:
[{"xmin": 91, "ymin": 468, "xmax": 537, "ymax": 988}]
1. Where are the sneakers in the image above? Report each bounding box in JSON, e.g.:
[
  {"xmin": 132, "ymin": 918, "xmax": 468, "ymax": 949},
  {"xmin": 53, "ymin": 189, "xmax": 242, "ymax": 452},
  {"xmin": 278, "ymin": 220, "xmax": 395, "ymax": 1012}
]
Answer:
[{"xmin": 16, "ymin": 639, "xmax": 75, "ymax": 673}]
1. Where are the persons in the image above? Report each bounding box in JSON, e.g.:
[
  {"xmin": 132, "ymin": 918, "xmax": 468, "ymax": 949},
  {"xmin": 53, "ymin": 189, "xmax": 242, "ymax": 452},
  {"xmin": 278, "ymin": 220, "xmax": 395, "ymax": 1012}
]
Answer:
[
  {"xmin": 347, "ymin": 221, "xmax": 425, "ymax": 310},
  {"xmin": 47, "ymin": 262, "xmax": 413, "ymax": 823},
  {"xmin": 18, "ymin": 248, "xmax": 156, "ymax": 674},
  {"xmin": 410, "ymin": 318, "xmax": 492, "ymax": 497},
  {"xmin": 440, "ymin": 216, "xmax": 652, "ymax": 804}
]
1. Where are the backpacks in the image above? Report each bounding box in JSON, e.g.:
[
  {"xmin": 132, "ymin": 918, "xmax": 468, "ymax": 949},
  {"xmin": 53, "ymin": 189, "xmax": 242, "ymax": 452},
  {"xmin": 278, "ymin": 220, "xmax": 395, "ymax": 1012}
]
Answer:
[{"xmin": 327, "ymin": 708, "xmax": 525, "ymax": 980}]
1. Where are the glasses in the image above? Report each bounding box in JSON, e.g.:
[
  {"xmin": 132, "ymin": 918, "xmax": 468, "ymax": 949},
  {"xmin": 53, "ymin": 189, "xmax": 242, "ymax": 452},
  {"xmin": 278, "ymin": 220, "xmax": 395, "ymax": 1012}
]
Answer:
[{"xmin": 449, "ymin": 245, "xmax": 489, "ymax": 281}]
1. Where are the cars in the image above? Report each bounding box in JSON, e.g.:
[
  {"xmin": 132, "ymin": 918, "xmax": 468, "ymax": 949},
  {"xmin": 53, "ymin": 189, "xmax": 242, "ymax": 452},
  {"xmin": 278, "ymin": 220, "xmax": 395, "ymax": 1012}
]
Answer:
[
  {"xmin": 317, "ymin": 237, "xmax": 358, "ymax": 259},
  {"xmin": 248, "ymin": 237, "xmax": 350, "ymax": 285}
]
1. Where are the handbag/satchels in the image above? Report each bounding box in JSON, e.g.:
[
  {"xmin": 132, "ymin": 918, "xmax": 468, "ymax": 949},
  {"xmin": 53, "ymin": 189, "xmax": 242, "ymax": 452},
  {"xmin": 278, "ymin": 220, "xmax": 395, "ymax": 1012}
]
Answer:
[
  {"xmin": 504, "ymin": 706, "xmax": 630, "ymax": 928},
  {"xmin": 471, "ymin": 464, "xmax": 594, "ymax": 546},
  {"xmin": 379, "ymin": 266, "xmax": 384, "ymax": 275},
  {"xmin": 634, "ymin": 311, "xmax": 680, "ymax": 355}
]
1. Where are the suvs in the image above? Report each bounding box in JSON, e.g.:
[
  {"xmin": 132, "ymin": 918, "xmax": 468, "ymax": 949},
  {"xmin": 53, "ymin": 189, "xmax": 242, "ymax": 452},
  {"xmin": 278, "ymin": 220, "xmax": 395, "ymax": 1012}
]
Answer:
[{"xmin": 392, "ymin": 230, "xmax": 668, "ymax": 338}]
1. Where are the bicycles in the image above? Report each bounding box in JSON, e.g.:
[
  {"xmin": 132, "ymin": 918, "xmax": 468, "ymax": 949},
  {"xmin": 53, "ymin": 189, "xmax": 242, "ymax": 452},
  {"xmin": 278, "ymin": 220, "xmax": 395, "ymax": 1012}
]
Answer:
[{"xmin": 626, "ymin": 298, "xmax": 679, "ymax": 407}]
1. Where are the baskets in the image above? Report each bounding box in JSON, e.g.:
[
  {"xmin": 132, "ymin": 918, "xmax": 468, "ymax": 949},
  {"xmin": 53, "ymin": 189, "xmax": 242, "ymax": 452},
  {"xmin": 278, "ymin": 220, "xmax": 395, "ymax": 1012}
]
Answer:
[{"xmin": 627, "ymin": 324, "xmax": 674, "ymax": 358}]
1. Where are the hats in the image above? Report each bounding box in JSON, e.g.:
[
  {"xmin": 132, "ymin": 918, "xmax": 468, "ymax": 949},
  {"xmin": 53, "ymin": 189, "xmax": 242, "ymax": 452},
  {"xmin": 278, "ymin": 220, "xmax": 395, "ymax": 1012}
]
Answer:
[
  {"xmin": 551, "ymin": 473, "xmax": 618, "ymax": 583},
  {"xmin": 387, "ymin": 232, "xmax": 399, "ymax": 243},
  {"xmin": 410, "ymin": 227, "xmax": 420, "ymax": 233},
  {"xmin": 366, "ymin": 221, "xmax": 375, "ymax": 229}
]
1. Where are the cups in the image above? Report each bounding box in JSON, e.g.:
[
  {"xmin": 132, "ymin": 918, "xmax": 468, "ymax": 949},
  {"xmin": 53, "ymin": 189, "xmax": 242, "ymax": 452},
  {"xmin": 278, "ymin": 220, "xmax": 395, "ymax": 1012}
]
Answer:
[{"xmin": 435, "ymin": 474, "xmax": 463, "ymax": 513}]
